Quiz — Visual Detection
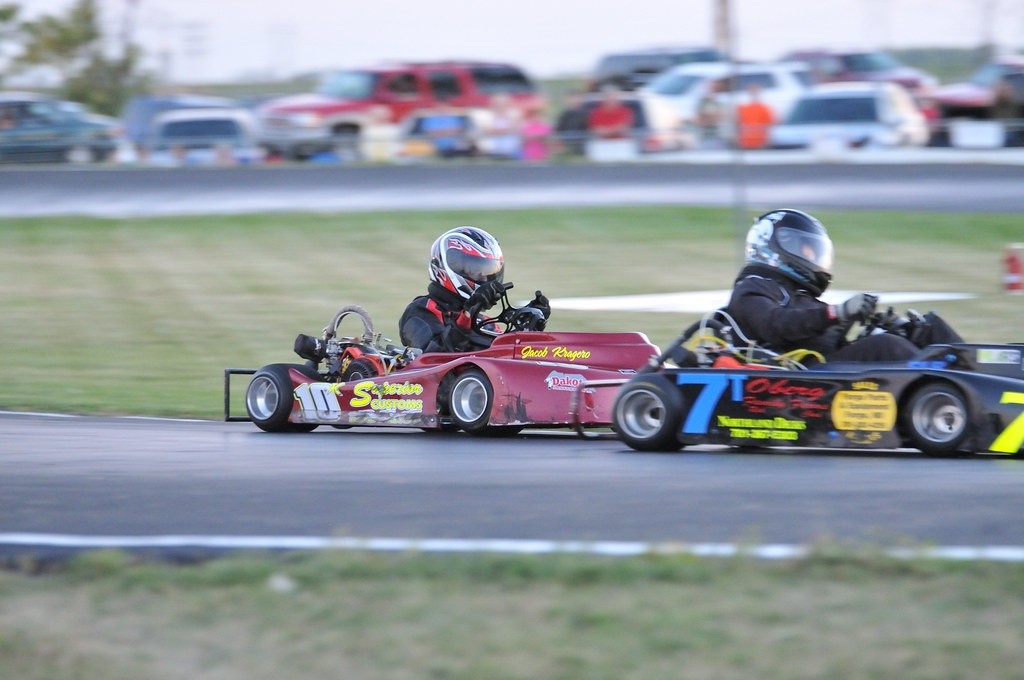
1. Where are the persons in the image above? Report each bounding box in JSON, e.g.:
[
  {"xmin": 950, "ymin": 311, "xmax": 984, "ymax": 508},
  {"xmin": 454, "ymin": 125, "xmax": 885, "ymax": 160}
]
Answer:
[
  {"xmin": 482, "ymin": 93, "xmax": 524, "ymax": 161},
  {"xmin": 398, "ymin": 226, "xmax": 551, "ymax": 353},
  {"xmin": 125, "ymin": 139, "xmax": 240, "ymax": 167},
  {"xmin": 879, "ymin": 78, "xmax": 929, "ymax": 148},
  {"xmin": 360, "ymin": 107, "xmax": 404, "ymax": 160},
  {"xmin": 728, "ymin": 208, "xmax": 976, "ymax": 368},
  {"xmin": 734, "ymin": 84, "xmax": 772, "ymax": 151},
  {"xmin": 697, "ymin": 79, "xmax": 726, "ymax": 150},
  {"xmin": 522, "ymin": 102, "xmax": 552, "ymax": 162},
  {"xmin": 421, "ymin": 98, "xmax": 480, "ymax": 158},
  {"xmin": 585, "ymin": 84, "xmax": 635, "ymax": 159}
]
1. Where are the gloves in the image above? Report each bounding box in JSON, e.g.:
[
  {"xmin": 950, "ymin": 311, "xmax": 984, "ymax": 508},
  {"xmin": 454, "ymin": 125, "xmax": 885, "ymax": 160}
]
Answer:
[
  {"xmin": 874, "ymin": 309, "xmax": 900, "ymax": 327},
  {"xmin": 525, "ymin": 290, "xmax": 551, "ymax": 320},
  {"xmin": 463, "ymin": 280, "xmax": 506, "ymax": 311},
  {"xmin": 842, "ymin": 293, "xmax": 879, "ymax": 321}
]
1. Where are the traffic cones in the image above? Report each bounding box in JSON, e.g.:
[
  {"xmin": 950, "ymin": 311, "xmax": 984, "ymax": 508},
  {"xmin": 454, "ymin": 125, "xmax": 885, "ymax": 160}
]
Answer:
[{"xmin": 1004, "ymin": 256, "xmax": 1024, "ymax": 297}]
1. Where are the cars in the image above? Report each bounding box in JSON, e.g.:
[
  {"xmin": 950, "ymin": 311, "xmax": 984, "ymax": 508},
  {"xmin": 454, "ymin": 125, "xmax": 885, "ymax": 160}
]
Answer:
[{"xmin": 0, "ymin": 49, "xmax": 1023, "ymax": 171}]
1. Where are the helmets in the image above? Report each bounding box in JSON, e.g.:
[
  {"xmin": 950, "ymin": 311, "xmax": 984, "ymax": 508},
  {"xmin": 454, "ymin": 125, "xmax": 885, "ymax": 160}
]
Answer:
[
  {"xmin": 743, "ymin": 208, "xmax": 833, "ymax": 297},
  {"xmin": 428, "ymin": 226, "xmax": 505, "ymax": 300}
]
